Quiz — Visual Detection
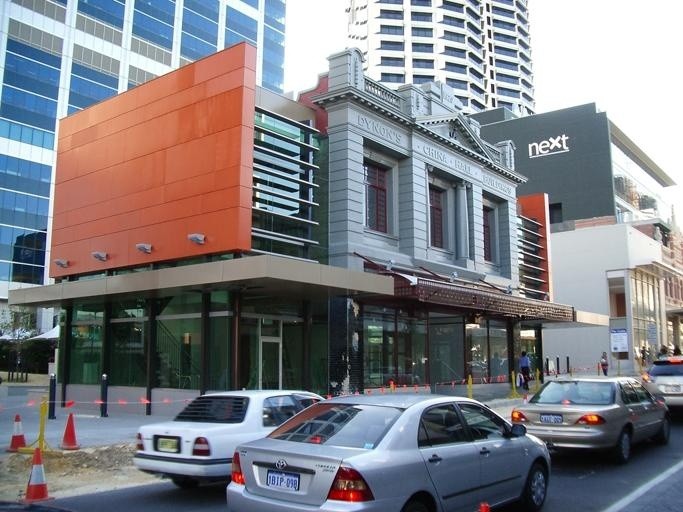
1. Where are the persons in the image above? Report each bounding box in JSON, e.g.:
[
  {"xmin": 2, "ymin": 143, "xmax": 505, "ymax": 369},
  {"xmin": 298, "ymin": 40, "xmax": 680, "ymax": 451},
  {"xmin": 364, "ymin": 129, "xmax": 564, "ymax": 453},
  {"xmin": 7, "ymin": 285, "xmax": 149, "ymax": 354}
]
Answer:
[
  {"xmin": 599, "ymin": 351, "xmax": 608, "ymax": 376},
  {"xmin": 515, "ymin": 350, "xmax": 531, "ymax": 390}
]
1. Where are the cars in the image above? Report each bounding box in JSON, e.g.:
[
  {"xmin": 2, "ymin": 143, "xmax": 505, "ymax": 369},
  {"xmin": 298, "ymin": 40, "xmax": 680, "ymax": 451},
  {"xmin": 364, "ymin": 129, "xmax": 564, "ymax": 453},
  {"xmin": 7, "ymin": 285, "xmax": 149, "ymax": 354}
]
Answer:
[
  {"xmin": 131, "ymin": 389, "xmax": 327, "ymax": 491},
  {"xmin": 467, "ymin": 358, "xmax": 510, "ymax": 378},
  {"xmin": 226, "ymin": 394, "xmax": 552, "ymax": 512},
  {"xmin": 511, "ymin": 355, "xmax": 683, "ymax": 463},
  {"xmin": 369, "ymin": 366, "xmax": 421, "ymax": 386}
]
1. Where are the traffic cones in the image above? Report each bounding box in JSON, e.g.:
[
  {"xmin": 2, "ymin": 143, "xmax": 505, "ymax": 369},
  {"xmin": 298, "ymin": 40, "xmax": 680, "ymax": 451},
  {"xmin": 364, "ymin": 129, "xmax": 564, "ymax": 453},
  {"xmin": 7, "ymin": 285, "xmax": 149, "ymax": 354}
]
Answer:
[
  {"xmin": 7, "ymin": 413, "xmax": 26, "ymax": 452},
  {"xmin": 22, "ymin": 448, "xmax": 55, "ymax": 503},
  {"xmin": 59, "ymin": 413, "xmax": 80, "ymax": 449}
]
